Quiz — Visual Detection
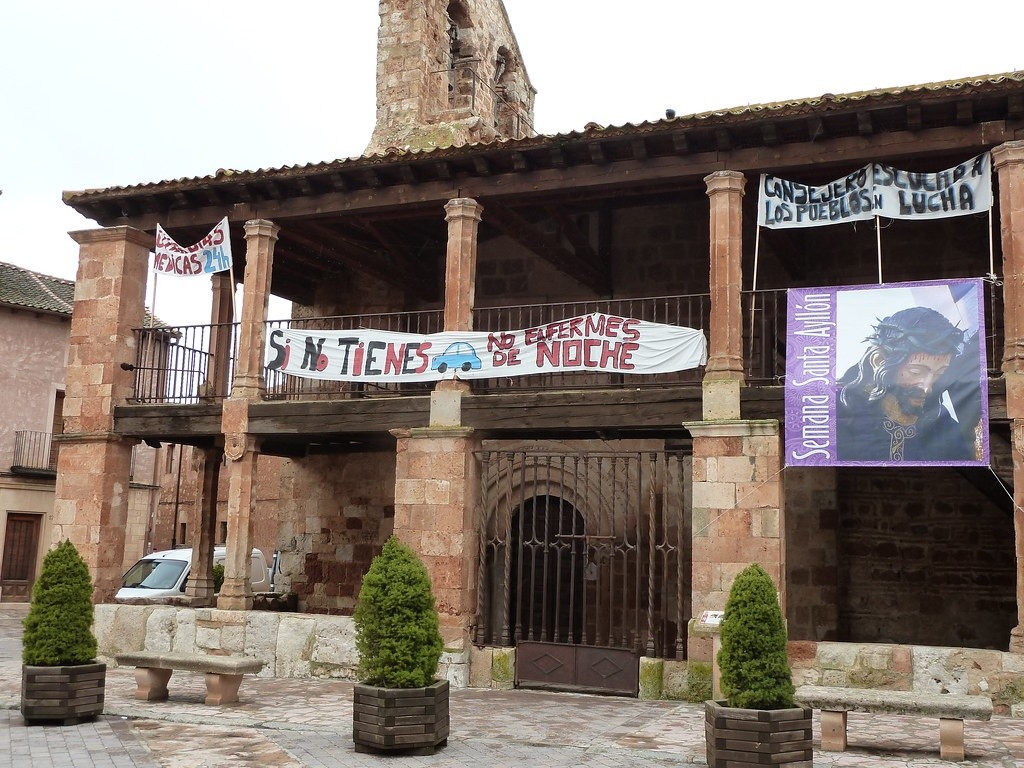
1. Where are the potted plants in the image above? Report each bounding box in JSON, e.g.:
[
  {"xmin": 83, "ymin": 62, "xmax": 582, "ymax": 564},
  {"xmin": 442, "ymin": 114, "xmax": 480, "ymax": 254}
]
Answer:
[
  {"xmin": 705, "ymin": 563, "xmax": 813, "ymax": 768},
  {"xmin": 21, "ymin": 538, "xmax": 106, "ymax": 725},
  {"xmin": 352, "ymin": 534, "xmax": 451, "ymax": 755}
]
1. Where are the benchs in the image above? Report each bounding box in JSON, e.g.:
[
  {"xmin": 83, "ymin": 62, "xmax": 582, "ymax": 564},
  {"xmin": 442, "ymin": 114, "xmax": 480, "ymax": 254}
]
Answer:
[
  {"xmin": 795, "ymin": 685, "xmax": 993, "ymax": 762},
  {"xmin": 115, "ymin": 651, "xmax": 265, "ymax": 706}
]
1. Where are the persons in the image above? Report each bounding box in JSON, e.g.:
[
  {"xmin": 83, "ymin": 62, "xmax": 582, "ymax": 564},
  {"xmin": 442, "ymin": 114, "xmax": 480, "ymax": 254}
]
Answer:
[{"xmin": 835, "ymin": 307, "xmax": 976, "ymax": 460}]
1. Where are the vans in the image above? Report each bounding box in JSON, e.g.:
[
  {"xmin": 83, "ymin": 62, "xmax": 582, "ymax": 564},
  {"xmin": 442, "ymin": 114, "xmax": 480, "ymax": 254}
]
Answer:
[{"xmin": 115, "ymin": 547, "xmax": 270, "ymax": 596}]
